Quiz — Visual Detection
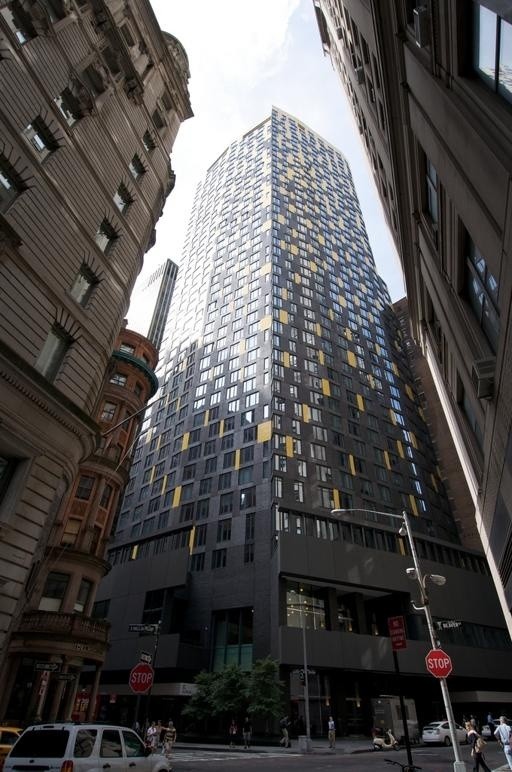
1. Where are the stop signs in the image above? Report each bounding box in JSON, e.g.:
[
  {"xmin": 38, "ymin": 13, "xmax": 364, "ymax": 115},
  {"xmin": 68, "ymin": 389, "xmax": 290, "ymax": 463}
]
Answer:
[
  {"xmin": 130, "ymin": 663, "xmax": 157, "ymax": 695},
  {"xmin": 425, "ymin": 649, "xmax": 455, "ymax": 680}
]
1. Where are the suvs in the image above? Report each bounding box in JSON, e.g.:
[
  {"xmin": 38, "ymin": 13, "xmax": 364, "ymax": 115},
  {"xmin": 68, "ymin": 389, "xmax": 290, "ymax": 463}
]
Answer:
[{"xmin": 3, "ymin": 721, "xmax": 171, "ymax": 772}]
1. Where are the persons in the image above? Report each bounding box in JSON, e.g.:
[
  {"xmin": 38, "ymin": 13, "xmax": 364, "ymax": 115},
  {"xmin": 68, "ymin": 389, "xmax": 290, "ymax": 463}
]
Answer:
[
  {"xmin": 229, "ymin": 719, "xmax": 237, "ymax": 747},
  {"xmin": 328, "ymin": 717, "xmax": 335, "ymax": 748},
  {"xmin": 466, "ymin": 712, "xmax": 512, "ymax": 772},
  {"xmin": 280, "ymin": 716, "xmax": 291, "ymax": 748},
  {"xmin": 133, "ymin": 719, "xmax": 176, "ymax": 759},
  {"xmin": 376, "ymin": 719, "xmax": 390, "ymax": 745},
  {"xmin": 242, "ymin": 718, "xmax": 252, "ymax": 749},
  {"xmin": 296, "ymin": 716, "xmax": 305, "ymax": 735}
]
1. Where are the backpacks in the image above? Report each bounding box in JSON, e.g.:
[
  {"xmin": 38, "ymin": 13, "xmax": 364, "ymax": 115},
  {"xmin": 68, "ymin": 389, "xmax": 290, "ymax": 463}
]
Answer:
[{"xmin": 474, "ymin": 737, "xmax": 488, "ymax": 753}]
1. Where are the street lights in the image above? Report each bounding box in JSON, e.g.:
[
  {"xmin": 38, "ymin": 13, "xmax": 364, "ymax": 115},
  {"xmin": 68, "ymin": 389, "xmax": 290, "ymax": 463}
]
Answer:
[
  {"xmin": 285, "ymin": 584, "xmax": 315, "ymax": 753},
  {"xmin": 327, "ymin": 504, "xmax": 469, "ymax": 770}
]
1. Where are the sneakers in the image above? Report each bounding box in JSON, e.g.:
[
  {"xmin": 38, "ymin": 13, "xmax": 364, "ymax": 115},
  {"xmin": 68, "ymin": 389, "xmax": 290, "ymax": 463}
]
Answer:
[{"xmin": 229, "ymin": 742, "xmax": 337, "ymax": 750}]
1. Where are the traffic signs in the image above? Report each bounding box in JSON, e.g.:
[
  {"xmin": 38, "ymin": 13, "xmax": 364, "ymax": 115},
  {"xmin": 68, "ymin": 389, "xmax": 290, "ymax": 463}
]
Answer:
[
  {"xmin": 137, "ymin": 651, "xmax": 154, "ymax": 664},
  {"xmin": 128, "ymin": 622, "xmax": 162, "ymax": 636}
]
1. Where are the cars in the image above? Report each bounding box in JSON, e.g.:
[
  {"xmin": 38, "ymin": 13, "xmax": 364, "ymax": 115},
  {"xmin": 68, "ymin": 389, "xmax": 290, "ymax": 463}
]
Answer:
[
  {"xmin": 0, "ymin": 726, "xmax": 23, "ymax": 767},
  {"xmin": 482, "ymin": 717, "xmax": 512, "ymax": 740},
  {"xmin": 419, "ymin": 720, "xmax": 471, "ymax": 746}
]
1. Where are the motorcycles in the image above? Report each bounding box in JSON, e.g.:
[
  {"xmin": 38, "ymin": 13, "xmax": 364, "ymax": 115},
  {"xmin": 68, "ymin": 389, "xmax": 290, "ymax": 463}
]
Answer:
[{"xmin": 370, "ymin": 727, "xmax": 401, "ymax": 753}]
